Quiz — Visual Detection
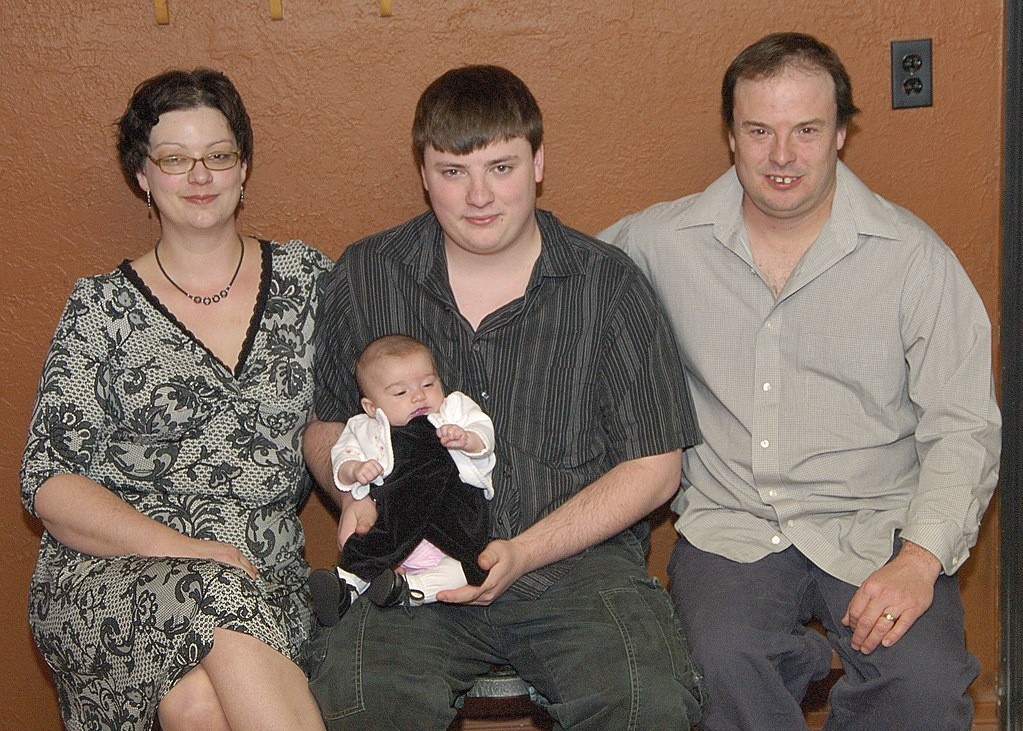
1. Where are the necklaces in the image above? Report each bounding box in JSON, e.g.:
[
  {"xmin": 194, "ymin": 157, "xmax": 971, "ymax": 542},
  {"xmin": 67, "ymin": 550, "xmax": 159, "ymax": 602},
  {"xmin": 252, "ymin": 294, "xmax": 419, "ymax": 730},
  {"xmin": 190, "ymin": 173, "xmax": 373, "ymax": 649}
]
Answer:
[{"xmin": 153, "ymin": 231, "xmax": 245, "ymax": 306}]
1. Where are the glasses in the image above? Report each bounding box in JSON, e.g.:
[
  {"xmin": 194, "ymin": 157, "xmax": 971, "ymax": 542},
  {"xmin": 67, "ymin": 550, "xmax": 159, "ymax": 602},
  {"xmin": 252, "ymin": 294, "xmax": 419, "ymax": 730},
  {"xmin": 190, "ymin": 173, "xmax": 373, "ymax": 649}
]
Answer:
[{"xmin": 147, "ymin": 150, "xmax": 242, "ymax": 176}]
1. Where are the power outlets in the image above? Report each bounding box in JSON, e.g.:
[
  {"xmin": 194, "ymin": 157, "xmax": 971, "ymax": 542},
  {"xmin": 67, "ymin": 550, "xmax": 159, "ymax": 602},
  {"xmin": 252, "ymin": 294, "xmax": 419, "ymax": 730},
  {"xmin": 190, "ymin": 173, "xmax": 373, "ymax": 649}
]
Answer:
[{"xmin": 891, "ymin": 37, "xmax": 933, "ymax": 109}]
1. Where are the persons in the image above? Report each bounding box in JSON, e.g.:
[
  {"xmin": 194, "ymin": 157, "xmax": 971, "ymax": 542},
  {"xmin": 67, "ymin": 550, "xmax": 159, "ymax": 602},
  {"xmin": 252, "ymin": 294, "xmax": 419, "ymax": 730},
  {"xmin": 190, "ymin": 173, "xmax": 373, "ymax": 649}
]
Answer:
[
  {"xmin": 313, "ymin": 65, "xmax": 706, "ymax": 731},
  {"xmin": 590, "ymin": 32, "xmax": 998, "ymax": 731},
  {"xmin": 306, "ymin": 336, "xmax": 500, "ymax": 630},
  {"xmin": 19, "ymin": 65, "xmax": 343, "ymax": 731}
]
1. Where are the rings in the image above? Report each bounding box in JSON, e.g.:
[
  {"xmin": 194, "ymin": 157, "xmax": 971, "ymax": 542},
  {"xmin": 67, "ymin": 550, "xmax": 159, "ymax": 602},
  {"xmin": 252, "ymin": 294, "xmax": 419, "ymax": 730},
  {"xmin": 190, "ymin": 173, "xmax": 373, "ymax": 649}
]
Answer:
[{"xmin": 880, "ymin": 613, "xmax": 895, "ymax": 622}]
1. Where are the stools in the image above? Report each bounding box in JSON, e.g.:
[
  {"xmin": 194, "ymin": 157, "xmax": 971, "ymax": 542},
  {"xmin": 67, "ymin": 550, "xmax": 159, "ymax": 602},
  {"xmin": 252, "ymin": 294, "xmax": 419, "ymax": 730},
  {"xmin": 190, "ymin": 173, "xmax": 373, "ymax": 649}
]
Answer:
[{"xmin": 447, "ymin": 617, "xmax": 844, "ymax": 731}]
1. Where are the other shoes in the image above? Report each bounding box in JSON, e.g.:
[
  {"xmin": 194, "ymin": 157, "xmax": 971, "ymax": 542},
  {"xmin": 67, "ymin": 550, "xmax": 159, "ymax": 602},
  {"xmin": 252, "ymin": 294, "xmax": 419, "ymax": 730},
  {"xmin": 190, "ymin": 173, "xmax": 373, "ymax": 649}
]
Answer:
[
  {"xmin": 368, "ymin": 569, "xmax": 425, "ymax": 608},
  {"xmin": 307, "ymin": 568, "xmax": 351, "ymax": 628}
]
